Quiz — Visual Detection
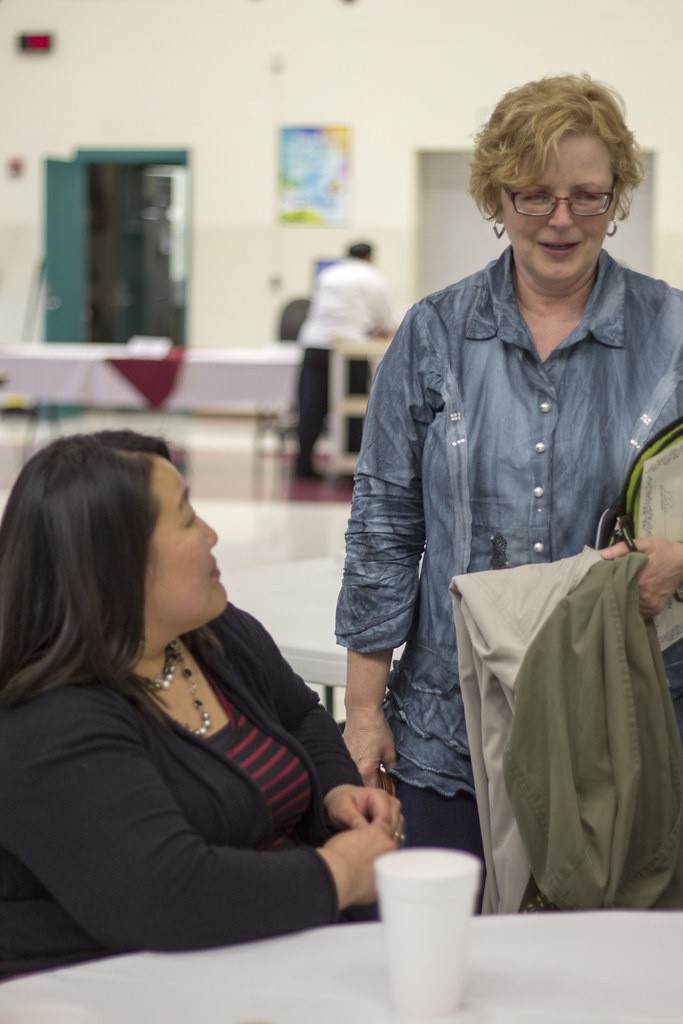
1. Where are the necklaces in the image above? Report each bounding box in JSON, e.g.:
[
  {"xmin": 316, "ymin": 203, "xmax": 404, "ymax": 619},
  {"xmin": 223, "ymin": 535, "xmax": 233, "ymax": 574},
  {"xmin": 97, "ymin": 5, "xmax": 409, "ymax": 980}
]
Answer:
[{"xmin": 131, "ymin": 637, "xmax": 212, "ymax": 738}]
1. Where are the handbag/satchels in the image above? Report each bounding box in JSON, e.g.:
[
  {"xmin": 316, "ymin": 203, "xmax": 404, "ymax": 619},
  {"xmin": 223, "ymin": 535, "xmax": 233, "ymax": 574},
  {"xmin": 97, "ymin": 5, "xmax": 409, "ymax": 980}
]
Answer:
[{"xmin": 596, "ymin": 417, "xmax": 683, "ymax": 553}]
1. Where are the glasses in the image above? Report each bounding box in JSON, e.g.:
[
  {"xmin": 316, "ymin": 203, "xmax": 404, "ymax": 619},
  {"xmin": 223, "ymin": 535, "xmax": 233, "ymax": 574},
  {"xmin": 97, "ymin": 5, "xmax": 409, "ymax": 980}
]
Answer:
[{"xmin": 502, "ymin": 175, "xmax": 617, "ymax": 217}]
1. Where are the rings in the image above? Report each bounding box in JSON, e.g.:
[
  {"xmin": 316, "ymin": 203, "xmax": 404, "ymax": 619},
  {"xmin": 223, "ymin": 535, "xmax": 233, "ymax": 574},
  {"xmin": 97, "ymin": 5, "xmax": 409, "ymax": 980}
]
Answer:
[{"xmin": 393, "ymin": 832, "xmax": 405, "ymax": 840}]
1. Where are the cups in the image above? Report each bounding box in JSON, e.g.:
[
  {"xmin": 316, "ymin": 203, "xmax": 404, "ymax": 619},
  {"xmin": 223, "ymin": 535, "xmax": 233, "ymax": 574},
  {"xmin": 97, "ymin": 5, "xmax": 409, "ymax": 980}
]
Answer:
[{"xmin": 375, "ymin": 847, "xmax": 484, "ymax": 1014}]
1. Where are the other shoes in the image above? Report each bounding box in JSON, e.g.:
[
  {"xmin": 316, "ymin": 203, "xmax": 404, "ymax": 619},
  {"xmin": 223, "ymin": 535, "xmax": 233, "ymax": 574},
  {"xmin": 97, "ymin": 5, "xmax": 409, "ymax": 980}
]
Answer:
[{"xmin": 296, "ymin": 458, "xmax": 323, "ymax": 479}]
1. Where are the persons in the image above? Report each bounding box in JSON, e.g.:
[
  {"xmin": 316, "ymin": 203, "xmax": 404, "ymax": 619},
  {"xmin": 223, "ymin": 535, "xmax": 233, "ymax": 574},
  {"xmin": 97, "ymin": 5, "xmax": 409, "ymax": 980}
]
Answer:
[
  {"xmin": 0, "ymin": 427, "xmax": 407, "ymax": 980},
  {"xmin": 291, "ymin": 239, "xmax": 402, "ymax": 491},
  {"xmin": 334, "ymin": 72, "xmax": 683, "ymax": 917}
]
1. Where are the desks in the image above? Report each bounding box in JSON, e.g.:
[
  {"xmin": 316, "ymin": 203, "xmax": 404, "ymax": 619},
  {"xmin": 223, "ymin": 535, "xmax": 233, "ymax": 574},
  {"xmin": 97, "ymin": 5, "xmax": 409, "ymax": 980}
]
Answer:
[
  {"xmin": 235, "ymin": 558, "xmax": 408, "ymax": 719},
  {"xmin": 0, "ymin": 342, "xmax": 306, "ymax": 416},
  {"xmin": 1, "ymin": 802, "xmax": 683, "ymax": 1024}
]
